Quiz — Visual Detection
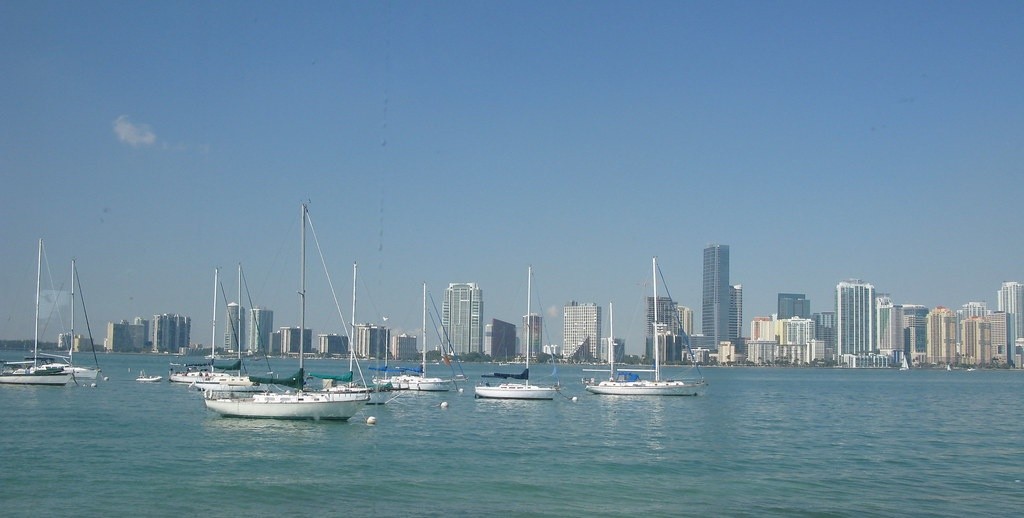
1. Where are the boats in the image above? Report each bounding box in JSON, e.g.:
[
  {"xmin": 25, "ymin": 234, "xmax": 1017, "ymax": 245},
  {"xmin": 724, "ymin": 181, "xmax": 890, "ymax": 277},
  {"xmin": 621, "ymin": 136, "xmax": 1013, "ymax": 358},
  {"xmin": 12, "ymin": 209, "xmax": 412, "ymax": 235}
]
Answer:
[{"xmin": 135, "ymin": 376, "xmax": 163, "ymax": 383}]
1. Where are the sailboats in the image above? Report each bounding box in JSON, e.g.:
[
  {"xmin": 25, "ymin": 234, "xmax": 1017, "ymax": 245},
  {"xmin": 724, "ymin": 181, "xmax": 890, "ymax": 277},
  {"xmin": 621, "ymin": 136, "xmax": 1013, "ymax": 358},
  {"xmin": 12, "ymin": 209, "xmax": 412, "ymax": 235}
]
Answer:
[
  {"xmin": 0, "ymin": 237, "xmax": 102, "ymax": 385},
  {"xmin": 581, "ymin": 255, "xmax": 708, "ymax": 397},
  {"xmin": 172, "ymin": 260, "xmax": 275, "ymax": 392},
  {"xmin": 369, "ymin": 282, "xmax": 454, "ymax": 392},
  {"xmin": 899, "ymin": 355, "xmax": 910, "ymax": 371},
  {"xmin": 473, "ymin": 264, "xmax": 564, "ymax": 400},
  {"xmin": 203, "ymin": 199, "xmax": 372, "ymax": 420}
]
